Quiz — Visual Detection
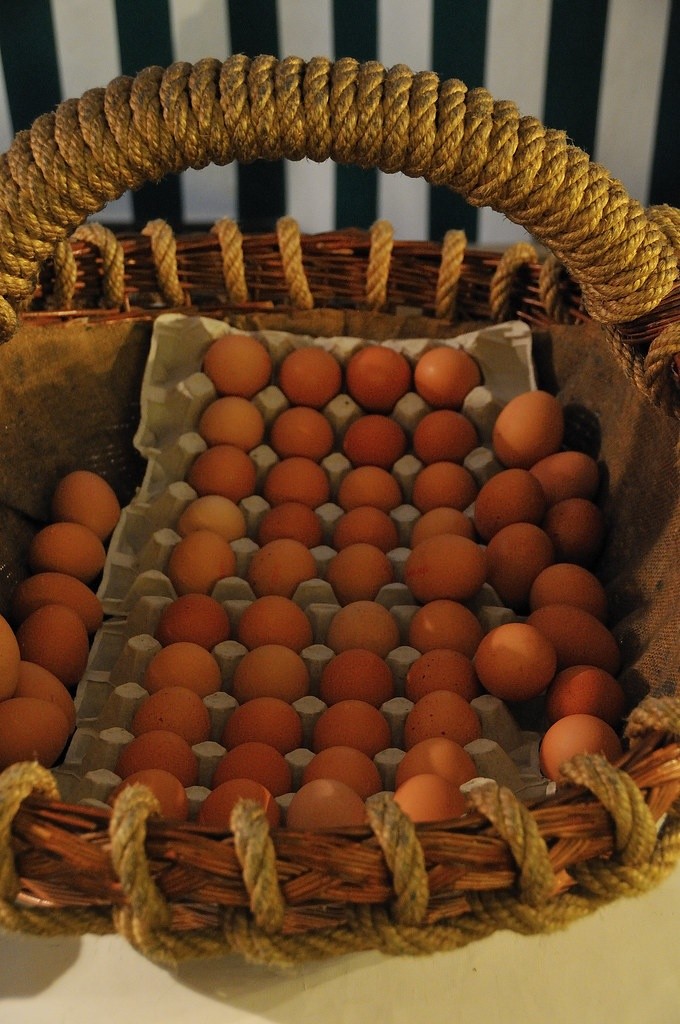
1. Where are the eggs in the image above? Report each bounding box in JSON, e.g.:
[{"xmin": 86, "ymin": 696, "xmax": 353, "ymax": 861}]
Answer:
[
  {"xmin": 100, "ymin": 332, "xmax": 622, "ymax": 829},
  {"xmin": 0, "ymin": 469, "xmax": 123, "ymax": 768}
]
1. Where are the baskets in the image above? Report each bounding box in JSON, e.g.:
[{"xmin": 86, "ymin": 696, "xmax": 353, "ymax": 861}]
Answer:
[{"xmin": 0, "ymin": 52, "xmax": 680, "ymax": 970}]
